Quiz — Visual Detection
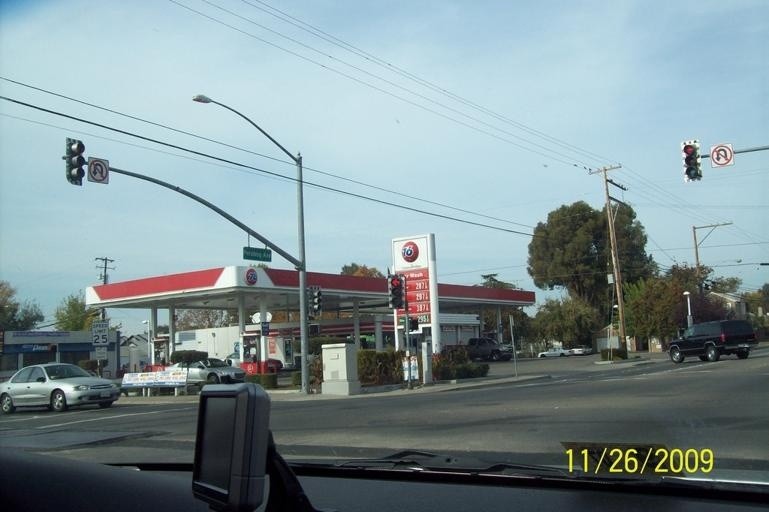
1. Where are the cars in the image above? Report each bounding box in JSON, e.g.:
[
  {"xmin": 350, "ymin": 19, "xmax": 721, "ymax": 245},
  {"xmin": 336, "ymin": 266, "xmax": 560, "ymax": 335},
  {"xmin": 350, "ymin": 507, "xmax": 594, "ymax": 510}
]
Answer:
[
  {"xmin": 537, "ymin": 346, "xmax": 573, "ymax": 358},
  {"xmin": 572, "ymin": 344, "xmax": 593, "ymax": 356},
  {"xmin": 144, "ymin": 349, "xmax": 284, "ymax": 387},
  {"xmin": 1, "ymin": 362, "xmax": 121, "ymax": 413}
]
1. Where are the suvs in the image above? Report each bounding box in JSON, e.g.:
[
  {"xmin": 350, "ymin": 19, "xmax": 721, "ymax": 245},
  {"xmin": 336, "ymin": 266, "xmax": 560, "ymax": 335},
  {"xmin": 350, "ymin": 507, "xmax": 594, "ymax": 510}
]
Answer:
[{"xmin": 665, "ymin": 319, "xmax": 758, "ymax": 364}]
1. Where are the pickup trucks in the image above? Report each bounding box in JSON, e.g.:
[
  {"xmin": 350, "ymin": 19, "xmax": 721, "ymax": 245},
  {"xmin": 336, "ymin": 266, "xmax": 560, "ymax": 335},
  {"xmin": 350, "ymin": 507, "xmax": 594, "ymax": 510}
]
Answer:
[{"xmin": 444, "ymin": 337, "xmax": 514, "ymax": 361}]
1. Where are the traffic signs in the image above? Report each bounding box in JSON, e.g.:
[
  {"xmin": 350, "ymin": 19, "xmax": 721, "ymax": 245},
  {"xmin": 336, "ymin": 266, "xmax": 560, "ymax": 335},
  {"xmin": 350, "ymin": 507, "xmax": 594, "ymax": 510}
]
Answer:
[
  {"xmin": 242, "ymin": 247, "xmax": 273, "ymax": 261},
  {"xmin": 92, "ymin": 322, "xmax": 109, "ymax": 347}
]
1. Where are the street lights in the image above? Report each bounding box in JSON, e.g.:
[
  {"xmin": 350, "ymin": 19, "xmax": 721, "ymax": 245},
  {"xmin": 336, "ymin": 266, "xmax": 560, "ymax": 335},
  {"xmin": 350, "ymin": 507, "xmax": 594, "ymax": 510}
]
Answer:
[
  {"xmin": 142, "ymin": 320, "xmax": 151, "ymax": 367},
  {"xmin": 193, "ymin": 95, "xmax": 311, "ymax": 393},
  {"xmin": 683, "ymin": 291, "xmax": 693, "ymax": 328}
]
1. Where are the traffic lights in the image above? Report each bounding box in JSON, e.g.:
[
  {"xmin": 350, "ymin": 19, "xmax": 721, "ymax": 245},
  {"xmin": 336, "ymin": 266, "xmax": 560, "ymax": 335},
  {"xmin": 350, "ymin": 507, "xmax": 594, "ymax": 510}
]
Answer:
[
  {"xmin": 388, "ymin": 276, "xmax": 405, "ymax": 310},
  {"xmin": 681, "ymin": 139, "xmax": 703, "ymax": 182},
  {"xmin": 312, "ymin": 290, "xmax": 322, "ymax": 312},
  {"xmin": 64, "ymin": 136, "xmax": 85, "ymax": 186}
]
